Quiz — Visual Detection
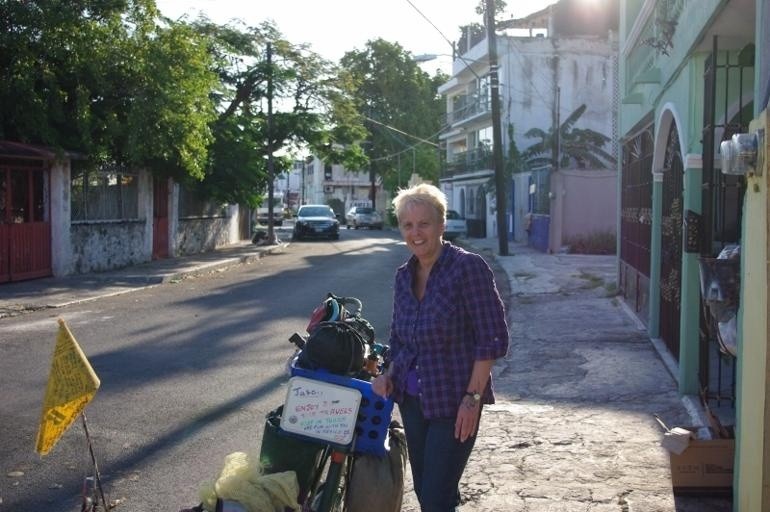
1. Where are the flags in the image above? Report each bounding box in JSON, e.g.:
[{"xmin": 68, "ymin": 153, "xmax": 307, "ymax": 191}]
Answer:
[{"xmin": 31, "ymin": 319, "xmax": 102, "ymax": 461}]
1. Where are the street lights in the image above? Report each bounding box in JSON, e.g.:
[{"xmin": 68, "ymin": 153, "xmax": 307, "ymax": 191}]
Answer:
[
  {"xmin": 263, "ymin": 54, "xmax": 307, "ymax": 246},
  {"xmin": 411, "ymin": 51, "xmax": 511, "ymax": 257}
]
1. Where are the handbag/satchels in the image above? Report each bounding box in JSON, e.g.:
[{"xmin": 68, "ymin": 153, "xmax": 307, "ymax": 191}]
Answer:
[{"xmin": 299, "ymin": 321, "xmax": 365, "ymax": 373}]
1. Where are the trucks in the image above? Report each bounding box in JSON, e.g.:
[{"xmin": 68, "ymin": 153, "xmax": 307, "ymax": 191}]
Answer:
[{"xmin": 255, "ymin": 191, "xmax": 287, "ymax": 226}]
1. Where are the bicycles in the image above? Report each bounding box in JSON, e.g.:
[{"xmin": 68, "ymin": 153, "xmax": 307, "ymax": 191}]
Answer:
[{"xmin": 276, "ymin": 288, "xmax": 399, "ymax": 512}]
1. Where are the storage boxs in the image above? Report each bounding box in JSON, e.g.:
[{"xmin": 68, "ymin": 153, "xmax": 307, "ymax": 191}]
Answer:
[{"xmin": 667, "ymin": 425, "xmax": 739, "ymax": 499}]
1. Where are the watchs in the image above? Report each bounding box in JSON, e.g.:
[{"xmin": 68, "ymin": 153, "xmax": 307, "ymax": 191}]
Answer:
[{"xmin": 462, "ymin": 388, "xmax": 484, "ymax": 403}]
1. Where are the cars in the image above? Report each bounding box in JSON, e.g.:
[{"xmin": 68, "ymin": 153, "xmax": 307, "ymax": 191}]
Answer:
[
  {"xmin": 441, "ymin": 209, "xmax": 469, "ymax": 244},
  {"xmin": 292, "ymin": 204, "xmax": 342, "ymax": 240},
  {"xmin": 343, "ymin": 207, "xmax": 386, "ymax": 230}
]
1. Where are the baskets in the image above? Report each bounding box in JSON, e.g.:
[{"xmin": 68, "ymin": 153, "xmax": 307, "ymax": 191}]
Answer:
[{"xmin": 277, "ymin": 356, "xmax": 393, "ymax": 457}]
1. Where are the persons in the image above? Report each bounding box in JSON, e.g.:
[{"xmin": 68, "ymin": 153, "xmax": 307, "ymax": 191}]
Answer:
[{"xmin": 369, "ymin": 181, "xmax": 512, "ymax": 512}]
